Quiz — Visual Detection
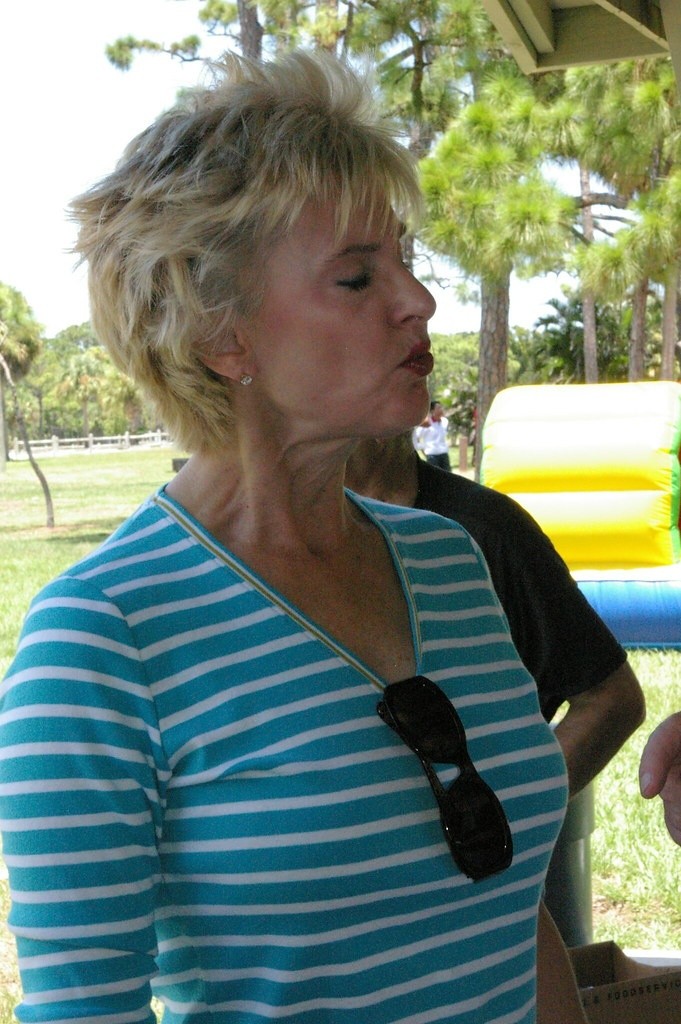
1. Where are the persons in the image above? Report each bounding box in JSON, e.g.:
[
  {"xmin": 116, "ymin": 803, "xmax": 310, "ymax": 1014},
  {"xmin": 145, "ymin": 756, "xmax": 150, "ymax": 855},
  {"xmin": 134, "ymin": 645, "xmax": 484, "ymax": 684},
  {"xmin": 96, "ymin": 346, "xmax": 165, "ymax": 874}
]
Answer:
[
  {"xmin": 0, "ymin": 45, "xmax": 587, "ymax": 1024},
  {"xmin": 414, "ymin": 402, "xmax": 451, "ymax": 473},
  {"xmin": 345, "ymin": 430, "xmax": 646, "ymax": 805},
  {"xmin": 640, "ymin": 711, "xmax": 681, "ymax": 846}
]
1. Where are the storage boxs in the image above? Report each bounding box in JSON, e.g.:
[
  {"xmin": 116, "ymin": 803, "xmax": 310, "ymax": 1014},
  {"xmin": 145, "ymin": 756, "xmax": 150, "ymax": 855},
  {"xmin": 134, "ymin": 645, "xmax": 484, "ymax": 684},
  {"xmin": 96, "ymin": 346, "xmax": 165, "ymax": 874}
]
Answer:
[{"xmin": 568, "ymin": 940, "xmax": 681, "ymax": 1024}]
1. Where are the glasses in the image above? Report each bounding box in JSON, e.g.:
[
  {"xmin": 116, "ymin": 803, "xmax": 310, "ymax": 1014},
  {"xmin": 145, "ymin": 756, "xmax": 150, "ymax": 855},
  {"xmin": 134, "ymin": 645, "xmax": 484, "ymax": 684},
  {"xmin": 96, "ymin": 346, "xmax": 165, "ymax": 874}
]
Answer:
[{"xmin": 375, "ymin": 675, "xmax": 514, "ymax": 884}]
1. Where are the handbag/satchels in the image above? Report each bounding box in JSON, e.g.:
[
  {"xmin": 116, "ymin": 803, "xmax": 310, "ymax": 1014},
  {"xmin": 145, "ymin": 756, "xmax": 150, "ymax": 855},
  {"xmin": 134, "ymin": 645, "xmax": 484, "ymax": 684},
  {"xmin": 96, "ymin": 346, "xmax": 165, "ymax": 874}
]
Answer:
[{"xmin": 536, "ymin": 900, "xmax": 681, "ymax": 1024}]
